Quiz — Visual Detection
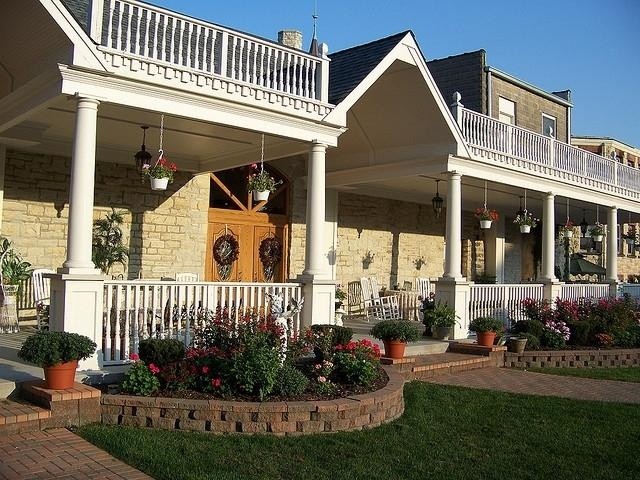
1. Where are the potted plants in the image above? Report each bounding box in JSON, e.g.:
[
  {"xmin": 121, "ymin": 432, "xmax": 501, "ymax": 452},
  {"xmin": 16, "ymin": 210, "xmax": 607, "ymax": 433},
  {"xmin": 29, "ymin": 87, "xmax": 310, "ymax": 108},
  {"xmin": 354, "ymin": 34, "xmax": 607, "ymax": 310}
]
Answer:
[
  {"xmin": 418, "ymin": 292, "xmax": 461, "ymax": 341},
  {"xmin": 17, "ymin": 329, "xmax": 97, "ymax": 390},
  {"xmin": 469, "ymin": 317, "xmax": 505, "ymax": 347}
]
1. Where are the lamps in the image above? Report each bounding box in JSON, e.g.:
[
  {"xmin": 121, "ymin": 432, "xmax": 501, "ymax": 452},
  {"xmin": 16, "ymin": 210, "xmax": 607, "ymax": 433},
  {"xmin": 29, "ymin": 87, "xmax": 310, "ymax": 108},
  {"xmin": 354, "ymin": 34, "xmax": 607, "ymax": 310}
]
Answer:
[
  {"xmin": 134, "ymin": 126, "xmax": 152, "ymax": 185},
  {"xmin": 432, "ymin": 179, "xmax": 443, "ymax": 218},
  {"xmin": 580, "ymin": 208, "xmax": 588, "ymax": 238}
]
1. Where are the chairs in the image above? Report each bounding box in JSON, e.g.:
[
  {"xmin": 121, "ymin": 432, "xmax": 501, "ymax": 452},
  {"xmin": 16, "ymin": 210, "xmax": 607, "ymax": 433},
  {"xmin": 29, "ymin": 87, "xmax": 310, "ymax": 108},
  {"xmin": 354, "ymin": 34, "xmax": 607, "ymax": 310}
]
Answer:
[
  {"xmin": 359, "ymin": 277, "xmax": 400, "ymax": 322},
  {"xmin": 32, "ymin": 268, "xmax": 55, "ymax": 331}
]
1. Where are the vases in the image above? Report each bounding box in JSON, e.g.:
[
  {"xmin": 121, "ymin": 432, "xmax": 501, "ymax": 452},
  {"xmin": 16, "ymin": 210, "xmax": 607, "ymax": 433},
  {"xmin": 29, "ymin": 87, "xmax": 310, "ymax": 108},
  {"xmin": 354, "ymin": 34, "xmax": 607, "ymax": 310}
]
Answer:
[
  {"xmin": 520, "ymin": 225, "xmax": 530, "ymax": 234},
  {"xmin": 253, "ymin": 189, "xmax": 270, "ymax": 201},
  {"xmin": 149, "ymin": 177, "xmax": 169, "ymax": 191},
  {"xmin": 510, "ymin": 336, "xmax": 528, "ymax": 353},
  {"xmin": 593, "ymin": 235, "xmax": 603, "ymax": 241},
  {"xmin": 626, "ymin": 238, "xmax": 634, "ymax": 244},
  {"xmin": 480, "ymin": 221, "xmax": 492, "ymax": 229},
  {"xmin": 565, "ymin": 231, "xmax": 572, "ymax": 237}
]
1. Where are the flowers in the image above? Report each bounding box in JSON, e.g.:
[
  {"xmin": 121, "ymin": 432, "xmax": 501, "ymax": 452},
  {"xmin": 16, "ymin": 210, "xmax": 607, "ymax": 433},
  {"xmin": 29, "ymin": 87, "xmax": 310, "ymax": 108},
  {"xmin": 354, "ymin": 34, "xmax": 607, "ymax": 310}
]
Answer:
[
  {"xmin": 246, "ymin": 164, "xmax": 284, "ymax": 194},
  {"xmin": 513, "ymin": 212, "xmax": 540, "ymax": 228},
  {"xmin": 473, "ymin": 208, "xmax": 498, "ymax": 221},
  {"xmin": 142, "ymin": 159, "xmax": 177, "ymax": 186}
]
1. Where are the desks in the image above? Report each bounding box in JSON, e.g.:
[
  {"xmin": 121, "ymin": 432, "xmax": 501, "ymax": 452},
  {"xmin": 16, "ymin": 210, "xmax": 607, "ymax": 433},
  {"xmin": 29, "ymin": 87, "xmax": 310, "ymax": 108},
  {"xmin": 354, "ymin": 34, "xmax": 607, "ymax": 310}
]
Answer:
[{"xmin": 386, "ymin": 288, "xmax": 420, "ymax": 321}]
